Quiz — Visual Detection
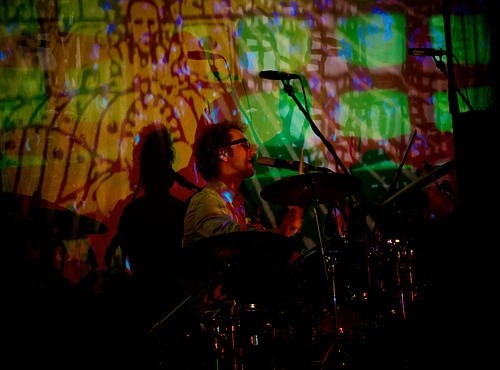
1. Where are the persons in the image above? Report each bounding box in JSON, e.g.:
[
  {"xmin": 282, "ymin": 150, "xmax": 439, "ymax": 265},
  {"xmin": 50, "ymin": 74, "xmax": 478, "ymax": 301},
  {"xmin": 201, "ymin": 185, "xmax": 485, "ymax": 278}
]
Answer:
[{"xmin": 183, "ymin": 123, "xmax": 303, "ymax": 246}]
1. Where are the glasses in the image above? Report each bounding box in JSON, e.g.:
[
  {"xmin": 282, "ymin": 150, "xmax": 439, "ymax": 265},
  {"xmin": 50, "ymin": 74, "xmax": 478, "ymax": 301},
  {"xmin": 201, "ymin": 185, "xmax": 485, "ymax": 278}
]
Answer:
[{"xmin": 220, "ymin": 137, "xmax": 250, "ymax": 149}]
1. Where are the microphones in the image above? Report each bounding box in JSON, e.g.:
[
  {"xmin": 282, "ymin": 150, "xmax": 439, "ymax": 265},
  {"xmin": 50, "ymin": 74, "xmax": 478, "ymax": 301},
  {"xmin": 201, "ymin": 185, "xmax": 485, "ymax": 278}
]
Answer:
[
  {"xmin": 407, "ymin": 47, "xmax": 444, "ymax": 55},
  {"xmin": 259, "ymin": 71, "xmax": 300, "ymax": 79},
  {"xmin": 257, "ymin": 156, "xmax": 316, "ymax": 172}
]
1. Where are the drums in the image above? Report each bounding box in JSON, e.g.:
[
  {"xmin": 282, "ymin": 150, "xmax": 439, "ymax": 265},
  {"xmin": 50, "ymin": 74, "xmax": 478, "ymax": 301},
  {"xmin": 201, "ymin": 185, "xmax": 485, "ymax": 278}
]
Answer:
[{"xmin": 199, "ymin": 234, "xmax": 418, "ymax": 370}]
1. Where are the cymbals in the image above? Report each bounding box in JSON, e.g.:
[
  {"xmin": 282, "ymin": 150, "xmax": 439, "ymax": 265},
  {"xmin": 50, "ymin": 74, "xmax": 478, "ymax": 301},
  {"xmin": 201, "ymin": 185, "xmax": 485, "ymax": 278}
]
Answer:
[
  {"xmin": 175, "ymin": 229, "xmax": 294, "ymax": 271},
  {"xmin": 0, "ymin": 191, "xmax": 75, "ymax": 214},
  {"xmin": 4, "ymin": 210, "xmax": 108, "ymax": 241},
  {"xmin": 259, "ymin": 172, "xmax": 363, "ymax": 207},
  {"xmin": 378, "ymin": 156, "xmax": 462, "ymax": 207}
]
